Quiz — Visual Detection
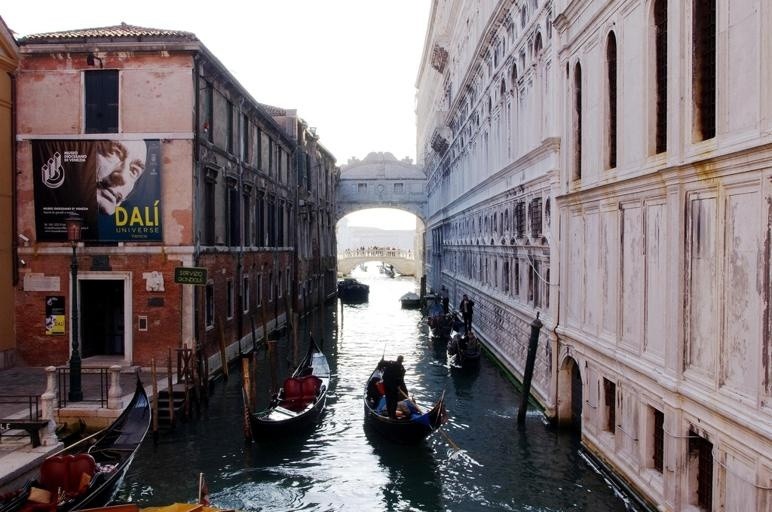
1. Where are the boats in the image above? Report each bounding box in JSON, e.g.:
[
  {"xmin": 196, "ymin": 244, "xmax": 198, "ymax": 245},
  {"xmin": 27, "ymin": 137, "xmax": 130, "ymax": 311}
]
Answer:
[
  {"xmin": 427, "ymin": 296, "xmax": 446, "ymax": 337},
  {"xmin": 337, "ymin": 277, "xmax": 370, "ymax": 301},
  {"xmin": 241, "ymin": 328, "xmax": 331, "ymax": 447},
  {"xmin": 0, "ymin": 370, "xmax": 153, "ymax": 512},
  {"xmin": 363, "ymin": 347, "xmax": 448, "ymax": 445},
  {"xmin": 359, "ymin": 263, "xmax": 396, "ymax": 278},
  {"xmin": 446, "ymin": 315, "xmax": 481, "ymax": 369},
  {"xmin": 400, "ymin": 291, "xmax": 420, "ymax": 308}
]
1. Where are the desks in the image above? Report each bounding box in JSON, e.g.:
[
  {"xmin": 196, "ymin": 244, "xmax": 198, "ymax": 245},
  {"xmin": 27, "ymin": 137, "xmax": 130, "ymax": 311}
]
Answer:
[{"xmin": 56, "ymin": 367, "xmax": 109, "ymax": 408}]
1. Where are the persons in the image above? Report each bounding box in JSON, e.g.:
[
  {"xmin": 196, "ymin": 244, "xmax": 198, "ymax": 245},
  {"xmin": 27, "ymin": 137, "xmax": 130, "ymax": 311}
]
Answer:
[
  {"xmin": 377, "ymin": 356, "xmax": 406, "ymax": 416},
  {"xmin": 367, "ymin": 377, "xmax": 382, "ymax": 409},
  {"xmin": 68, "ymin": 140, "xmax": 147, "ymax": 216},
  {"xmin": 438, "ymin": 285, "xmax": 449, "ymax": 314},
  {"xmin": 459, "ymin": 294, "xmax": 474, "ymax": 335}
]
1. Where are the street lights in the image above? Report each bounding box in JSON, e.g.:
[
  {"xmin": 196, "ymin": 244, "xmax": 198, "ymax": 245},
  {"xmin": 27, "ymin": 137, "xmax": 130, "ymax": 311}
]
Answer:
[{"xmin": 64, "ymin": 215, "xmax": 84, "ymax": 401}]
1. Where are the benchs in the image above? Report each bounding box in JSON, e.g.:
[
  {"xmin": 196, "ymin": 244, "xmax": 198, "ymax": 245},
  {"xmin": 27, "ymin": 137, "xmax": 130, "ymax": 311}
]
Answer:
[{"xmin": 0, "ymin": 419, "xmax": 50, "ymax": 448}]
